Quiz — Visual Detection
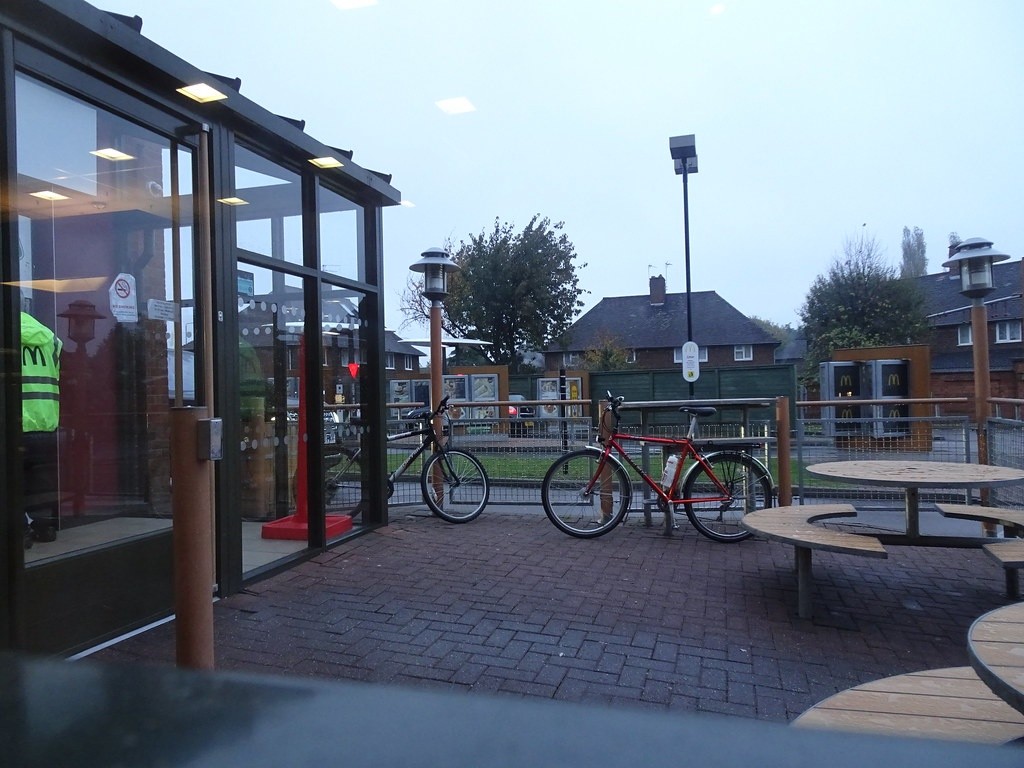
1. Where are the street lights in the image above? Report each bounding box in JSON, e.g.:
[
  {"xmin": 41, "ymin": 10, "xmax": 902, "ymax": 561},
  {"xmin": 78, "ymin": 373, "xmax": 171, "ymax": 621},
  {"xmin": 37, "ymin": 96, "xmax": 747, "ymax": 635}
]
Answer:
[
  {"xmin": 940, "ymin": 237, "xmax": 1014, "ymax": 538},
  {"xmin": 408, "ymin": 245, "xmax": 465, "ymax": 515}
]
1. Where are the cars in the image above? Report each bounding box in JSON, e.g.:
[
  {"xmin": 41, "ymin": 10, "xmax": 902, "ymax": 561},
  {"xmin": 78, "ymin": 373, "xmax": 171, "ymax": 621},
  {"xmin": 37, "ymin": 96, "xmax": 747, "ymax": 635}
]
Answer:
[
  {"xmin": 508, "ymin": 393, "xmax": 536, "ymax": 437},
  {"xmin": 407, "ymin": 404, "xmax": 433, "ymax": 433}
]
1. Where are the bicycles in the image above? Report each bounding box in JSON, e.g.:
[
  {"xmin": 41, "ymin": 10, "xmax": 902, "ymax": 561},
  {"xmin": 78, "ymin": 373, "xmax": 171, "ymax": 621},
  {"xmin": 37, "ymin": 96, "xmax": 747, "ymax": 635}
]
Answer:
[
  {"xmin": 539, "ymin": 388, "xmax": 775, "ymax": 543},
  {"xmin": 289, "ymin": 393, "xmax": 491, "ymax": 524}
]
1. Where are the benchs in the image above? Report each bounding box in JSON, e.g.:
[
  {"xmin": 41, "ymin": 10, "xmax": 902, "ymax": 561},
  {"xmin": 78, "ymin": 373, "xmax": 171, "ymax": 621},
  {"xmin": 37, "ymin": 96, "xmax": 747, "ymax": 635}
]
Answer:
[
  {"xmin": 790, "ymin": 666, "xmax": 1024, "ymax": 749},
  {"xmin": 741, "ymin": 502, "xmax": 889, "ymax": 627},
  {"xmin": 934, "ymin": 503, "xmax": 1024, "ymax": 600}
]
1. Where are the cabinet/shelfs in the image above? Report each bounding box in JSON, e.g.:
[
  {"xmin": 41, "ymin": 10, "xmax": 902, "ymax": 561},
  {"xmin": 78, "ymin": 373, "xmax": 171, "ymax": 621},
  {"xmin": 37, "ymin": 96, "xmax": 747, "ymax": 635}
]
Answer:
[{"xmin": 241, "ymin": 422, "xmax": 300, "ymax": 523}]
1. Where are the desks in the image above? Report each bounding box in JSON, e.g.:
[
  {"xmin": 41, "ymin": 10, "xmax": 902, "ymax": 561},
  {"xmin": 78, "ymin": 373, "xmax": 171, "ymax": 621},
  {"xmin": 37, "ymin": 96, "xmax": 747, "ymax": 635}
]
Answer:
[
  {"xmin": 964, "ymin": 601, "xmax": 1024, "ymax": 712},
  {"xmin": 806, "ymin": 460, "xmax": 1024, "ymax": 536}
]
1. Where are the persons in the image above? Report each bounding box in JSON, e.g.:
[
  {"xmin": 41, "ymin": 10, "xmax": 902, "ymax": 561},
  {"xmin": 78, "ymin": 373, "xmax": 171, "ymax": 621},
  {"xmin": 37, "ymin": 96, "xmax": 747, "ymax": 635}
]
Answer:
[{"xmin": 21, "ymin": 310, "xmax": 65, "ymax": 551}]
